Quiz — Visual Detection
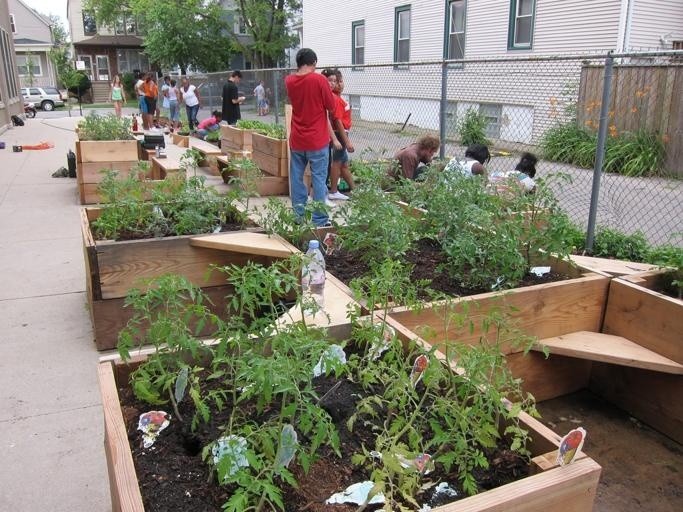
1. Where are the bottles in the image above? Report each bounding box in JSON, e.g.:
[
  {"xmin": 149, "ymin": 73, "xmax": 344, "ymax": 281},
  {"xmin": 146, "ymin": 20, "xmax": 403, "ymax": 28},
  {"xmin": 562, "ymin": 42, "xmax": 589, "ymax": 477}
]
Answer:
[
  {"xmin": 131, "ymin": 115, "xmax": 137, "ymax": 131},
  {"xmin": 300, "ymin": 238, "xmax": 324, "ymax": 318}
]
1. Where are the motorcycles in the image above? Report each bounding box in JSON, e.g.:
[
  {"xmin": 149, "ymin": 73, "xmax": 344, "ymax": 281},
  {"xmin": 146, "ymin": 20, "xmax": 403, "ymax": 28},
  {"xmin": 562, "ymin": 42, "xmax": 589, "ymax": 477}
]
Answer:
[{"xmin": 22, "ymin": 103, "xmax": 36, "ymax": 118}]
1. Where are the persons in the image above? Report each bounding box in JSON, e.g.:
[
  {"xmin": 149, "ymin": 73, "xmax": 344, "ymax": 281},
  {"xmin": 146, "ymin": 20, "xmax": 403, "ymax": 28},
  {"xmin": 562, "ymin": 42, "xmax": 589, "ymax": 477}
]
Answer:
[
  {"xmin": 134, "ymin": 69, "xmax": 247, "ymax": 141},
  {"xmin": 253, "ymin": 80, "xmax": 281, "ymax": 116},
  {"xmin": 109, "ymin": 75, "xmax": 127, "ymax": 120},
  {"xmin": 307, "ymin": 67, "xmax": 343, "ymax": 203},
  {"xmin": 484, "ymin": 152, "xmax": 539, "ymax": 201},
  {"xmin": 285, "ymin": 48, "xmax": 337, "ymax": 227},
  {"xmin": 328, "ymin": 67, "xmax": 363, "ymax": 200},
  {"xmin": 381, "ymin": 135, "xmax": 438, "ymax": 190},
  {"xmin": 442, "ymin": 144, "xmax": 490, "ymax": 197}
]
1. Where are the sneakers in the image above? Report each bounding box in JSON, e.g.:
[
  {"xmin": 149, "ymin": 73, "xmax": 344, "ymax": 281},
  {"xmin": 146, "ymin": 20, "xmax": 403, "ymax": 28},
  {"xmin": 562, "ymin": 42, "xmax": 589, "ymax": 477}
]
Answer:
[{"xmin": 328, "ymin": 191, "xmax": 349, "ymax": 200}]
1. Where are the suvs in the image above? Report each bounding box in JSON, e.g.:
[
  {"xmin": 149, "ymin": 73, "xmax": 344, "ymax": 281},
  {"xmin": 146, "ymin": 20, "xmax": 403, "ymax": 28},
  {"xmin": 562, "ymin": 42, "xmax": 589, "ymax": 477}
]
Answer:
[{"xmin": 20, "ymin": 87, "xmax": 63, "ymax": 111}]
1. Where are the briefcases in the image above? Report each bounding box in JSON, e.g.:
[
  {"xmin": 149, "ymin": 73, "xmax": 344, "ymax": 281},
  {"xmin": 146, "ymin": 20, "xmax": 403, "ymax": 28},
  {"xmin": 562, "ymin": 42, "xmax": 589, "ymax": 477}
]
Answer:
[{"xmin": 67, "ymin": 149, "xmax": 75, "ymax": 177}]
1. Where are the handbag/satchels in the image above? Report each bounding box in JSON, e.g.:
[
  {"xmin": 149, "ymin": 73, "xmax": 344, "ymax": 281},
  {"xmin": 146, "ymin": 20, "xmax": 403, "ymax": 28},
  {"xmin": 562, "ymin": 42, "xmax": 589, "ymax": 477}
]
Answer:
[
  {"xmin": 141, "ymin": 141, "xmax": 165, "ymax": 149},
  {"xmin": 11, "ymin": 115, "xmax": 24, "ymax": 126}
]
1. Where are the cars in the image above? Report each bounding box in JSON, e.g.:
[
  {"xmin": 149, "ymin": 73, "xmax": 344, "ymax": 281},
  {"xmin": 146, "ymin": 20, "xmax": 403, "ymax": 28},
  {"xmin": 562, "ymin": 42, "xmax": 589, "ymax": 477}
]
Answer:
[
  {"xmin": 59, "ymin": 90, "xmax": 67, "ymax": 101},
  {"xmin": 190, "ymin": 81, "xmax": 246, "ymax": 107}
]
